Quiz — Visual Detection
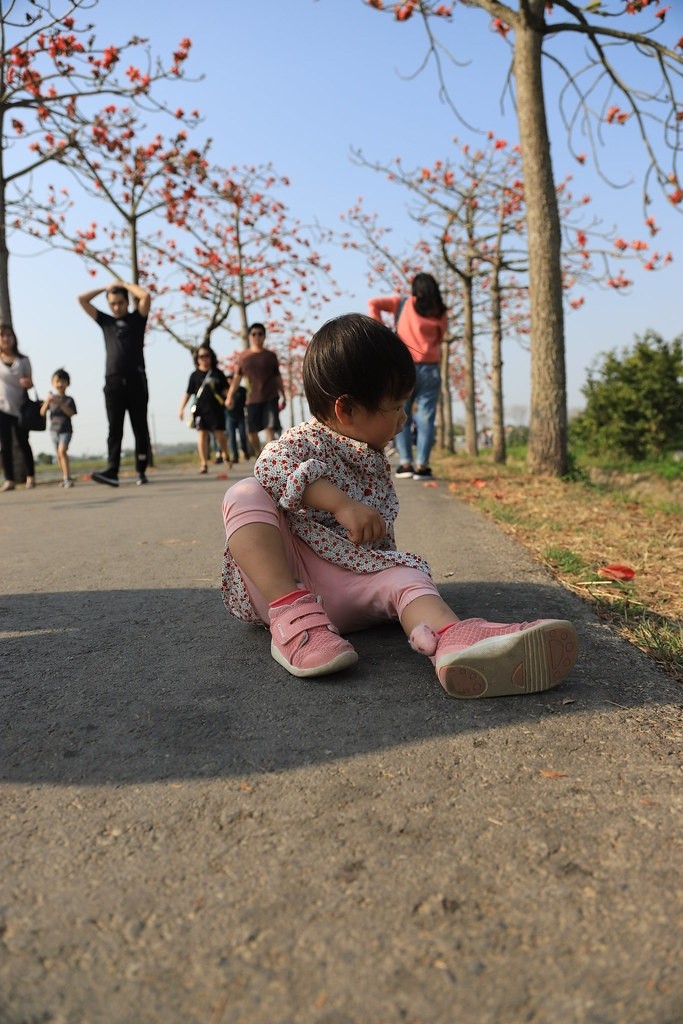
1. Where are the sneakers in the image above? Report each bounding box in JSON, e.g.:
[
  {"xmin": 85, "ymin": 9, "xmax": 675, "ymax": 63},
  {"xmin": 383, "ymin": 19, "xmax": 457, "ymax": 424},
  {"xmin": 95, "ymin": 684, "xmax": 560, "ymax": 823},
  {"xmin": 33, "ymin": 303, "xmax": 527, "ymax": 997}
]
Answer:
[
  {"xmin": 268, "ymin": 594, "xmax": 359, "ymax": 678},
  {"xmin": 395, "ymin": 466, "xmax": 415, "ymax": 478},
  {"xmin": 413, "ymin": 468, "xmax": 432, "ymax": 480},
  {"xmin": 137, "ymin": 471, "xmax": 148, "ymax": 486},
  {"xmin": 91, "ymin": 469, "xmax": 119, "ymax": 486},
  {"xmin": 428, "ymin": 617, "xmax": 580, "ymax": 699}
]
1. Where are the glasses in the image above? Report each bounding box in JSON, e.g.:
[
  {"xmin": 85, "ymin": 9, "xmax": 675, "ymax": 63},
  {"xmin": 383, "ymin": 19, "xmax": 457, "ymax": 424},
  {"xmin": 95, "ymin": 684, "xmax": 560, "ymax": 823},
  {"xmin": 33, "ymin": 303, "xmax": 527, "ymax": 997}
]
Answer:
[
  {"xmin": 252, "ymin": 332, "xmax": 263, "ymax": 337},
  {"xmin": 200, "ymin": 355, "xmax": 210, "ymax": 360}
]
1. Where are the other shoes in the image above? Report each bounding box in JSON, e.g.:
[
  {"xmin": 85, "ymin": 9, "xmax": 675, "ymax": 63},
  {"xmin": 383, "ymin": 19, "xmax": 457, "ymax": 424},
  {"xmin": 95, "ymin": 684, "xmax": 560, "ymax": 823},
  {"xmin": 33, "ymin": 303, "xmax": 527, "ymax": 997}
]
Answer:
[
  {"xmin": 26, "ymin": 483, "xmax": 35, "ymax": 489},
  {"xmin": 200, "ymin": 464, "xmax": 207, "ymax": 473},
  {"xmin": 58, "ymin": 478, "xmax": 72, "ymax": 488},
  {"xmin": 243, "ymin": 449, "xmax": 251, "ymax": 459},
  {"xmin": 223, "ymin": 456, "xmax": 232, "ymax": 469},
  {"xmin": 0, "ymin": 484, "xmax": 14, "ymax": 492},
  {"xmin": 233, "ymin": 456, "xmax": 238, "ymax": 462}
]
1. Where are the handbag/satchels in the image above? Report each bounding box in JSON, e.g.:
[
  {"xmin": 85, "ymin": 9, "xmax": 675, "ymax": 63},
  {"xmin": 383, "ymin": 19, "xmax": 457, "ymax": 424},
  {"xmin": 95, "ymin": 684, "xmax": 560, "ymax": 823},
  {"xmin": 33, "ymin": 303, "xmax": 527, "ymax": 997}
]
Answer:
[
  {"xmin": 18, "ymin": 384, "xmax": 46, "ymax": 431},
  {"xmin": 188, "ymin": 404, "xmax": 197, "ymax": 428}
]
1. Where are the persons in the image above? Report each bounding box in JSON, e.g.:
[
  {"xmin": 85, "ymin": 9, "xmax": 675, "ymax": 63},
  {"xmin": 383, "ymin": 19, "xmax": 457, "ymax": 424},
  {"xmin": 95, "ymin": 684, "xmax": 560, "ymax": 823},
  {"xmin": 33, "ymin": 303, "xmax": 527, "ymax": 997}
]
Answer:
[
  {"xmin": 221, "ymin": 313, "xmax": 579, "ymax": 698},
  {"xmin": 0, "ymin": 323, "xmax": 35, "ymax": 490},
  {"xmin": 222, "ymin": 322, "xmax": 286, "ymax": 456},
  {"xmin": 77, "ymin": 282, "xmax": 154, "ymax": 487},
  {"xmin": 176, "ymin": 344, "xmax": 233, "ymax": 472},
  {"xmin": 369, "ymin": 273, "xmax": 448, "ymax": 480},
  {"xmin": 40, "ymin": 368, "xmax": 77, "ymax": 488},
  {"xmin": 223, "ymin": 372, "xmax": 251, "ymax": 463}
]
